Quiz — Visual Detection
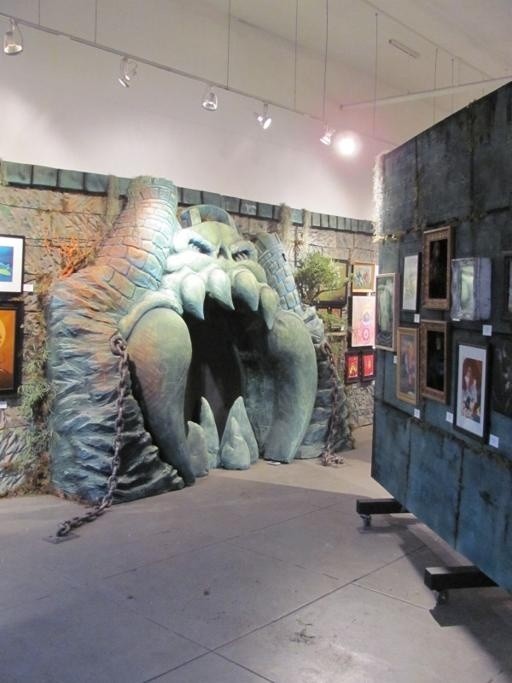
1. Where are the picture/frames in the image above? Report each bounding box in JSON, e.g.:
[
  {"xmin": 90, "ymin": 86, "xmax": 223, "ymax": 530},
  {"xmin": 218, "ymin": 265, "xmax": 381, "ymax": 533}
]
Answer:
[
  {"xmin": 399, "ymin": 252, "xmax": 422, "ymax": 313},
  {"xmin": 0, "ymin": 301, "xmax": 24, "ymax": 399},
  {"xmin": 315, "ymin": 259, "xmax": 376, "ymax": 383},
  {"xmin": 396, "ymin": 327, "xmax": 420, "ymax": 406},
  {"xmin": 375, "ymin": 273, "xmax": 398, "ymax": 353},
  {"xmin": 0, "ymin": 234, "xmax": 25, "ymax": 294},
  {"xmin": 422, "ymin": 227, "xmax": 512, "ymax": 443}
]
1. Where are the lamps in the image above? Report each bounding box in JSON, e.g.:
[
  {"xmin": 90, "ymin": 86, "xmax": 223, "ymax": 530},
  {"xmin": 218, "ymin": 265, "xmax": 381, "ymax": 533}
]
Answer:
[
  {"xmin": 2, "ymin": 18, "xmax": 23, "ymax": 56},
  {"xmin": 256, "ymin": 104, "xmax": 272, "ymax": 129},
  {"xmin": 319, "ymin": 125, "xmax": 335, "ymax": 145},
  {"xmin": 201, "ymin": 85, "xmax": 217, "ymax": 111},
  {"xmin": 118, "ymin": 56, "xmax": 138, "ymax": 89}
]
